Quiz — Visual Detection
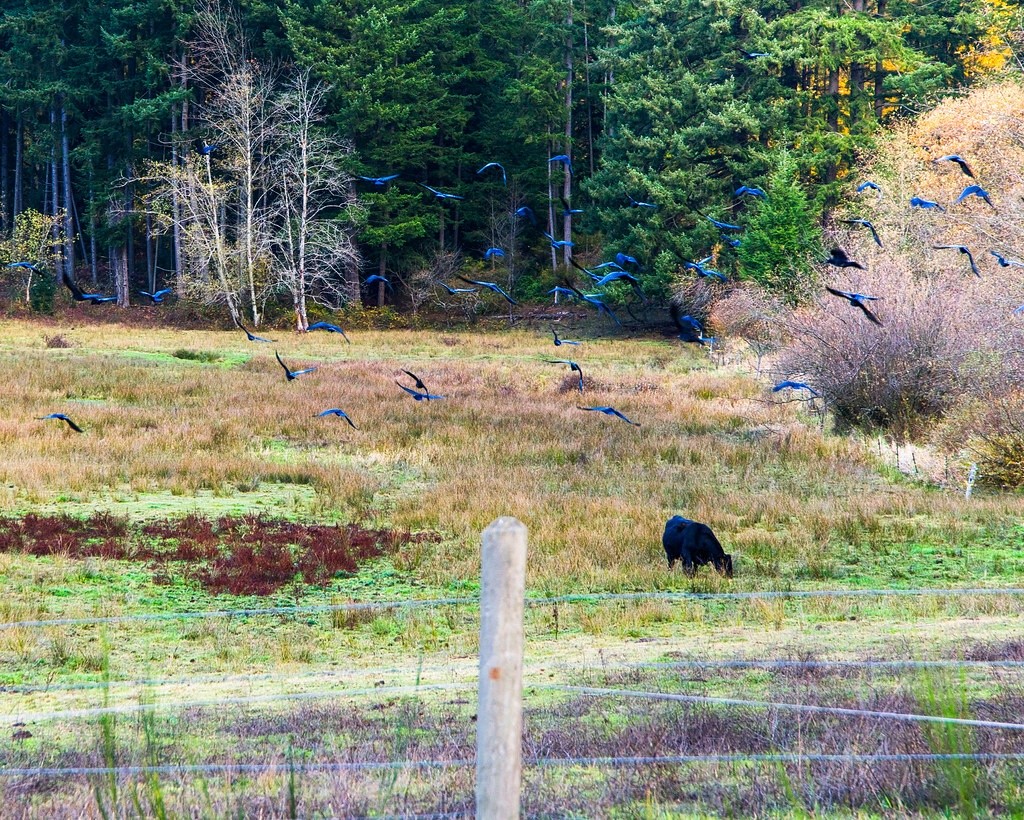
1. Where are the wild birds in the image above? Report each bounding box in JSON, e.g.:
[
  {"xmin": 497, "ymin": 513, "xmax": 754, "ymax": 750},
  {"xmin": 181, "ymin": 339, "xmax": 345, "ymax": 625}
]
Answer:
[{"xmin": 2, "ymin": 46, "xmax": 1024, "ymax": 435}]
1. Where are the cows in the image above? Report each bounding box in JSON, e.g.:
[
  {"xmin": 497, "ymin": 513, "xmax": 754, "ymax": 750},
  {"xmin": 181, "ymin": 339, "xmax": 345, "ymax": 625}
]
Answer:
[{"xmin": 663, "ymin": 515, "xmax": 732, "ymax": 578}]
